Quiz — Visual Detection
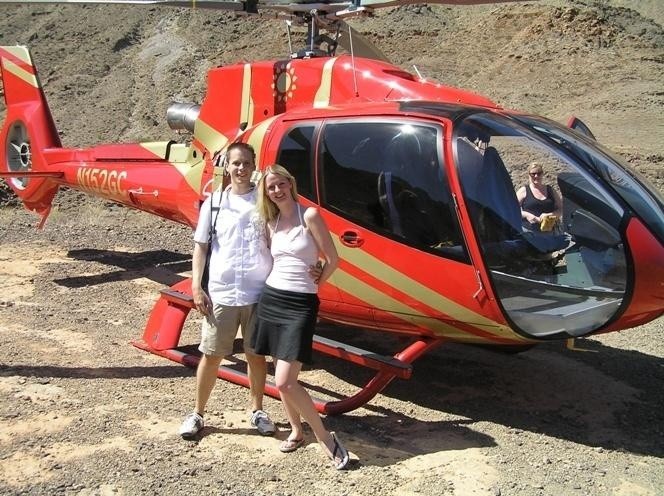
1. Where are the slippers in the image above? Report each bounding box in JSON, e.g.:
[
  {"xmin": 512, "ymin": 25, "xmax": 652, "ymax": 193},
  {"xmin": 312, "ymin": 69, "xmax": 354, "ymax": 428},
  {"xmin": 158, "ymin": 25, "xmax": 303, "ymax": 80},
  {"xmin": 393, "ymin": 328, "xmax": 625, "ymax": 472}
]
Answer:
[
  {"xmin": 279, "ymin": 433, "xmax": 306, "ymax": 453},
  {"xmin": 321, "ymin": 430, "xmax": 349, "ymax": 470}
]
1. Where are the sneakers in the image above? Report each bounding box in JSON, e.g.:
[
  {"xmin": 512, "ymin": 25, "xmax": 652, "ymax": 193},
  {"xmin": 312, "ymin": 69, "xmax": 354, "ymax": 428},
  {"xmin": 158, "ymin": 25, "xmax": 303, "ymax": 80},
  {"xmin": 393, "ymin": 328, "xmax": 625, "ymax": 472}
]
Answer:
[
  {"xmin": 179, "ymin": 412, "xmax": 205, "ymax": 437},
  {"xmin": 249, "ymin": 407, "xmax": 275, "ymax": 437}
]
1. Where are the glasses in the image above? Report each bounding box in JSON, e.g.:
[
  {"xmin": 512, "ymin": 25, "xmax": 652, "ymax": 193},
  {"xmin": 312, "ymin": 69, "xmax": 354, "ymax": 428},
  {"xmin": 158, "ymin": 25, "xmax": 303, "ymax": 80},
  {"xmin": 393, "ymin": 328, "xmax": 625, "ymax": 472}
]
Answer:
[{"xmin": 529, "ymin": 172, "xmax": 543, "ymax": 177}]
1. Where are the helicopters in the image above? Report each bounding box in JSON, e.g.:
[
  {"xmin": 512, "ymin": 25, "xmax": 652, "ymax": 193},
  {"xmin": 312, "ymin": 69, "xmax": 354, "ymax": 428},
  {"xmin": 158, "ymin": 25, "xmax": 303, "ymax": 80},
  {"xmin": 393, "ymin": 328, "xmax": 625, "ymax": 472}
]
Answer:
[{"xmin": 2, "ymin": 0, "xmax": 663, "ymax": 414}]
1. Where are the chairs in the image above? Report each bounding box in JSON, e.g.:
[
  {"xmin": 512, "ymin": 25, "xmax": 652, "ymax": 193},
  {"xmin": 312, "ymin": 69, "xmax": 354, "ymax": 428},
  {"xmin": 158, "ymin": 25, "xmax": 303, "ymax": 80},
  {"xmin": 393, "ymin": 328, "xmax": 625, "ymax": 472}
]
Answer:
[{"xmin": 386, "ymin": 146, "xmax": 570, "ymax": 258}]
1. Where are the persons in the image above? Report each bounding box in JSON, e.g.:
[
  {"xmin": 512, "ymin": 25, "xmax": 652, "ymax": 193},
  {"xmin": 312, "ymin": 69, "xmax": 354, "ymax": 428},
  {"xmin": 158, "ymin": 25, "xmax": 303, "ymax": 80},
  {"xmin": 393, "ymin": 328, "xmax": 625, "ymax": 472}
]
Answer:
[
  {"xmin": 250, "ymin": 164, "xmax": 349, "ymax": 471},
  {"xmin": 516, "ymin": 162, "xmax": 562, "ymax": 237},
  {"xmin": 179, "ymin": 142, "xmax": 323, "ymax": 439}
]
